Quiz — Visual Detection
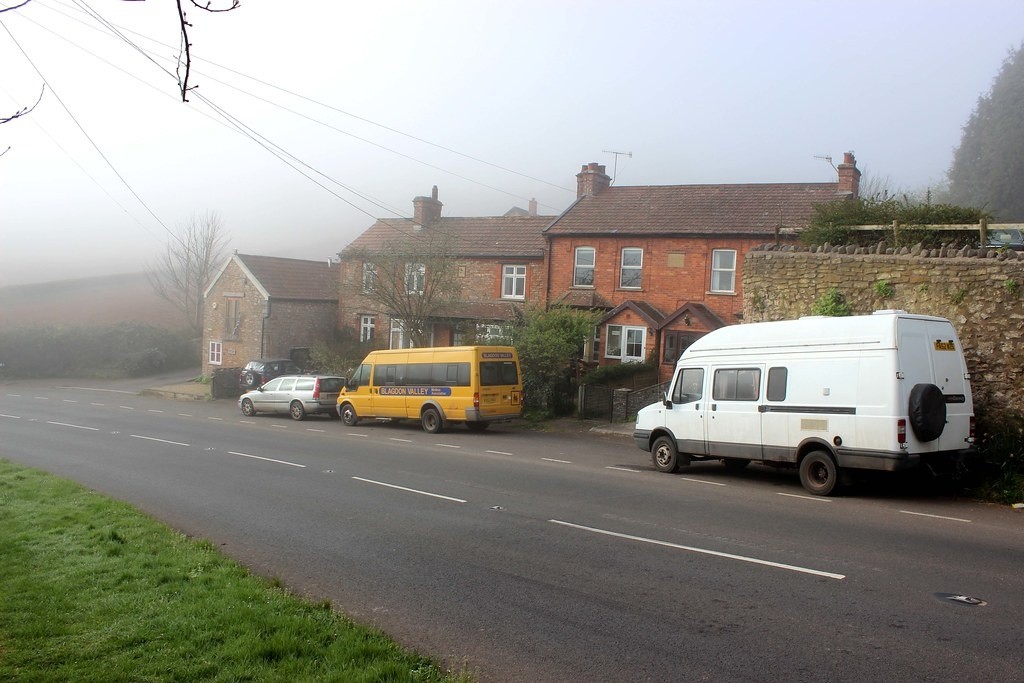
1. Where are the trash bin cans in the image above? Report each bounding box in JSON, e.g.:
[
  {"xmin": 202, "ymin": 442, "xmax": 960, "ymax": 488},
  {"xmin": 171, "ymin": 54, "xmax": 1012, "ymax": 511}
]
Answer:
[{"xmin": 210, "ymin": 368, "xmax": 245, "ymax": 399}]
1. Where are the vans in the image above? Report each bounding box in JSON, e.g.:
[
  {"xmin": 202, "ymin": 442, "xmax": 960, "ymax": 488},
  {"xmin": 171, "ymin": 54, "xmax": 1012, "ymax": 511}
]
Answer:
[
  {"xmin": 632, "ymin": 309, "xmax": 982, "ymax": 497},
  {"xmin": 334, "ymin": 344, "xmax": 526, "ymax": 434}
]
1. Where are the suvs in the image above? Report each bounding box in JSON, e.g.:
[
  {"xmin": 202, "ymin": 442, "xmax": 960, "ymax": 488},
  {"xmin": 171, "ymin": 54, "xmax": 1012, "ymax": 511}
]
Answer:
[
  {"xmin": 239, "ymin": 357, "xmax": 311, "ymax": 394},
  {"xmin": 238, "ymin": 373, "xmax": 346, "ymax": 421}
]
